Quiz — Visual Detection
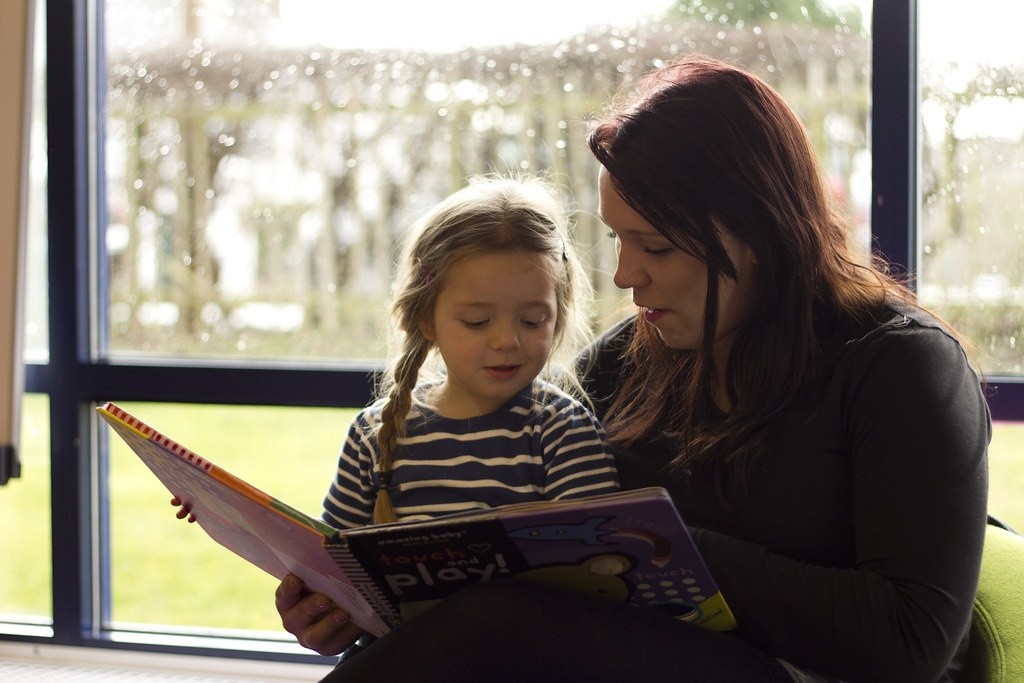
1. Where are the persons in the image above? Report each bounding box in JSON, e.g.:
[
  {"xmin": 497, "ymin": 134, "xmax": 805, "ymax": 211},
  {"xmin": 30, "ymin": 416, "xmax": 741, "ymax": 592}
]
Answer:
[
  {"xmin": 277, "ymin": 62, "xmax": 992, "ymax": 683},
  {"xmin": 170, "ymin": 179, "xmax": 619, "ymax": 617}
]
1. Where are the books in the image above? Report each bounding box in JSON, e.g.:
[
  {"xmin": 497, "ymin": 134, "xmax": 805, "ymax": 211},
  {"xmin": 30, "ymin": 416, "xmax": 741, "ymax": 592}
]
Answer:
[{"xmin": 96, "ymin": 403, "xmax": 737, "ymax": 640}]
